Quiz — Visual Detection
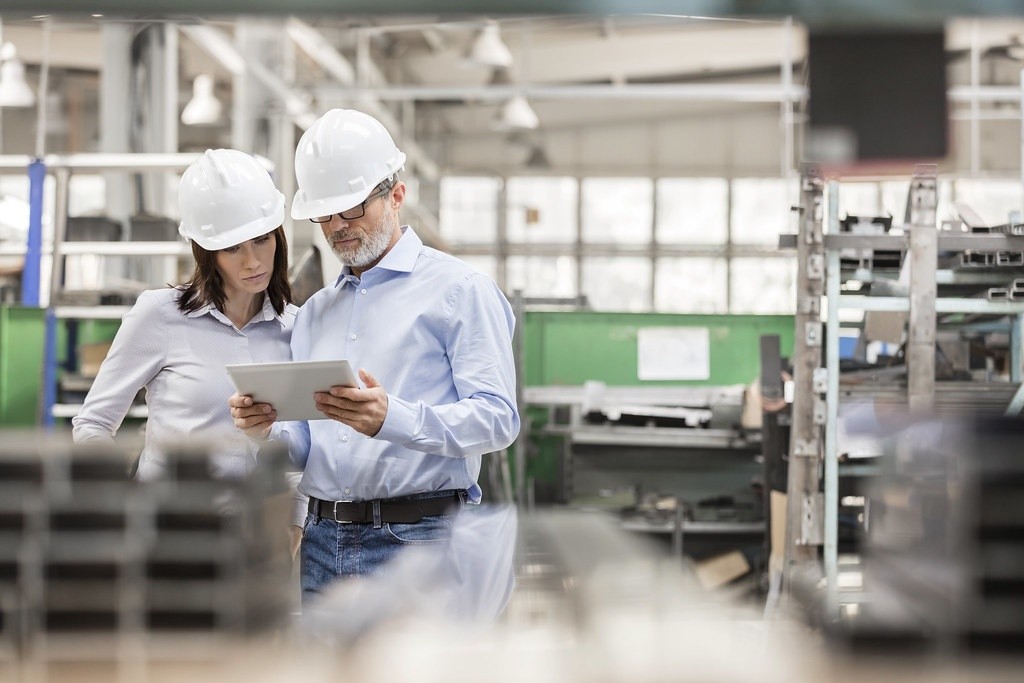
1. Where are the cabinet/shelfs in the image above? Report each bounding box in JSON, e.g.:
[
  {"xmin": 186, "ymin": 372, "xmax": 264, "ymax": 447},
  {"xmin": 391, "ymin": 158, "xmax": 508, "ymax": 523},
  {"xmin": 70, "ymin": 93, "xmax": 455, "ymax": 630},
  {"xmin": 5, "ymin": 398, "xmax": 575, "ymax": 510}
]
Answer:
[
  {"xmin": 0, "ymin": 153, "xmax": 204, "ymax": 420},
  {"xmin": 561, "ymin": 434, "xmax": 768, "ymax": 618}
]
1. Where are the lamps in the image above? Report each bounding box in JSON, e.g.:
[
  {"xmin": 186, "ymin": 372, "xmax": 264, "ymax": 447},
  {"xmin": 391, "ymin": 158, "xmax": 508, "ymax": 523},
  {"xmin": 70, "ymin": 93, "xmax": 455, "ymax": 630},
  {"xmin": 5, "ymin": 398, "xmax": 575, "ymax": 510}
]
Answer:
[
  {"xmin": 460, "ymin": 19, "xmax": 515, "ymax": 67},
  {"xmin": 484, "ymin": 87, "xmax": 540, "ymax": 130},
  {"xmin": 179, "ymin": 72, "xmax": 222, "ymax": 125},
  {"xmin": 0, "ymin": 41, "xmax": 35, "ymax": 106}
]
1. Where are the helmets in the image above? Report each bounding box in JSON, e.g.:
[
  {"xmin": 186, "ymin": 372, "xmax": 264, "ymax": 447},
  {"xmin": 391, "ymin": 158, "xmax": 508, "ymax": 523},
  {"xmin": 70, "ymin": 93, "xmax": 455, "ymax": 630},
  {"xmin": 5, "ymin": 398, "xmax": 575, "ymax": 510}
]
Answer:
[
  {"xmin": 290, "ymin": 108, "xmax": 406, "ymax": 220},
  {"xmin": 178, "ymin": 148, "xmax": 287, "ymax": 251}
]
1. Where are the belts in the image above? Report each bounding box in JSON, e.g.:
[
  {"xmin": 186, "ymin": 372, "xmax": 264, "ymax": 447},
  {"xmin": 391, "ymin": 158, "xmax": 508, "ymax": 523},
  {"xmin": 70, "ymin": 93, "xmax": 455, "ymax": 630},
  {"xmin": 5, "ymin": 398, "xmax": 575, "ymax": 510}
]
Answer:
[{"xmin": 308, "ymin": 494, "xmax": 467, "ymax": 523}]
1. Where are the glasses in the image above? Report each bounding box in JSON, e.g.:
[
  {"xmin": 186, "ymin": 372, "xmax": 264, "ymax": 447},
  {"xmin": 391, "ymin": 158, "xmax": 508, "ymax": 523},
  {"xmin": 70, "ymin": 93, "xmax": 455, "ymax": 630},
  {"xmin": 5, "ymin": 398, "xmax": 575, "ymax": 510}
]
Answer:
[{"xmin": 309, "ymin": 185, "xmax": 391, "ymax": 223}]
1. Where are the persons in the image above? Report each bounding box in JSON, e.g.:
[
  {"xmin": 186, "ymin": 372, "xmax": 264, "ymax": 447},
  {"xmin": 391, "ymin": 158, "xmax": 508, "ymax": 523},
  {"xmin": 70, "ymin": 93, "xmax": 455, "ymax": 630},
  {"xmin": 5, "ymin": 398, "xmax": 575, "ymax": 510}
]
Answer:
[
  {"xmin": 230, "ymin": 108, "xmax": 521, "ymax": 627},
  {"xmin": 72, "ymin": 148, "xmax": 310, "ymax": 628}
]
